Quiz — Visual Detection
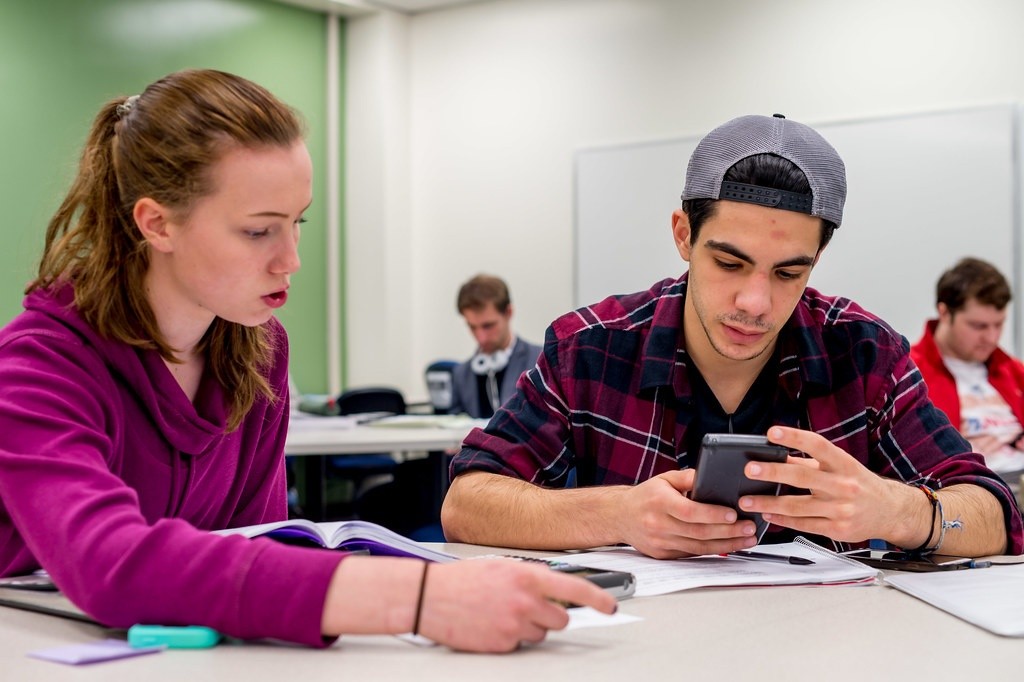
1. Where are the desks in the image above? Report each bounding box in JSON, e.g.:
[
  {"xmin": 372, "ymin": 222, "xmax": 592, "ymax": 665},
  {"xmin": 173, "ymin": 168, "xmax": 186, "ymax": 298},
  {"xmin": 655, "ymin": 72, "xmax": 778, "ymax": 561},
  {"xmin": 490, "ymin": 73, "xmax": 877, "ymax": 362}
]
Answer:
[
  {"xmin": 0, "ymin": 541, "xmax": 1024, "ymax": 682},
  {"xmin": 285, "ymin": 408, "xmax": 493, "ymax": 453}
]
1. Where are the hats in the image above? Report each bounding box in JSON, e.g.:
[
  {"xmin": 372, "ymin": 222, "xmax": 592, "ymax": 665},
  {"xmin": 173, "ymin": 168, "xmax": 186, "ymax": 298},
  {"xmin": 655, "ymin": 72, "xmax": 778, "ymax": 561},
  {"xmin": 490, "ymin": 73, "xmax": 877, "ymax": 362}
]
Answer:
[{"xmin": 681, "ymin": 113, "xmax": 848, "ymax": 229}]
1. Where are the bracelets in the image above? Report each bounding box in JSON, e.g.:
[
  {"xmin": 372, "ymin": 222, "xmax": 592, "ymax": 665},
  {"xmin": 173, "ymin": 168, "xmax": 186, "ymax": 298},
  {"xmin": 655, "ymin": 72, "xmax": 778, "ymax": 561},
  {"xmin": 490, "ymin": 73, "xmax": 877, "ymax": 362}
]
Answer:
[
  {"xmin": 906, "ymin": 477, "xmax": 960, "ymax": 557},
  {"xmin": 414, "ymin": 555, "xmax": 432, "ymax": 635}
]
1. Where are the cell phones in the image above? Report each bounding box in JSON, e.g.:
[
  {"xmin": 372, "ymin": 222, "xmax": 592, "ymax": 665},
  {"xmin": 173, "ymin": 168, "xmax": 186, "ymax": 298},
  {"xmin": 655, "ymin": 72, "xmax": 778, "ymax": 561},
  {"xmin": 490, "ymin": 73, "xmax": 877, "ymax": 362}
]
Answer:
[
  {"xmin": 127, "ymin": 623, "xmax": 222, "ymax": 649},
  {"xmin": 690, "ymin": 433, "xmax": 788, "ymax": 546}
]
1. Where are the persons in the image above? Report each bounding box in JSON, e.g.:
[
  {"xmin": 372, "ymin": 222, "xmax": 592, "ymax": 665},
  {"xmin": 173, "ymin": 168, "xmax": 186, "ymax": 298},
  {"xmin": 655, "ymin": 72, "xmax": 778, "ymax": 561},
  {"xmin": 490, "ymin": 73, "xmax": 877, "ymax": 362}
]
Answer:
[
  {"xmin": 441, "ymin": 113, "xmax": 1024, "ymax": 558},
  {"xmin": 446, "ymin": 276, "xmax": 576, "ymax": 490},
  {"xmin": 0, "ymin": 70, "xmax": 619, "ymax": 654},
  {"xmin": 906, "ymin": 257, "xmax": 1024, "ymax": 515}
]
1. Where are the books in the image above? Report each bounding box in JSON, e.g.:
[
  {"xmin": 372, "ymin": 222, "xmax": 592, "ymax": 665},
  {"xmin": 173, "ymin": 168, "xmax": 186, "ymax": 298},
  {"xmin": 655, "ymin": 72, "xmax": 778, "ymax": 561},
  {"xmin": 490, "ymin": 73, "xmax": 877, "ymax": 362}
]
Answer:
[
  {"xmin": 550, "ymin": 536, "xmax": 884, "ymax": 598},
  {"xmin": 192, "ymin": 518, "xmax": 460, "ymax": 564}
]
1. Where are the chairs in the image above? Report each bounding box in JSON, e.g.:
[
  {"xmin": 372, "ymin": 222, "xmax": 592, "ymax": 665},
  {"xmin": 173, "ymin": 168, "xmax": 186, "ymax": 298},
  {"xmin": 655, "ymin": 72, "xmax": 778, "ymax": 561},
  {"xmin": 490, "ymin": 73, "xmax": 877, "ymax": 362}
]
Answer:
[
  {"xmin": 319, "ymin": 389, "xmax": 408, "ymax": 500},
  {"xmin": 425, "ymin": 360, "xmax": 462, "ymax": 415}
]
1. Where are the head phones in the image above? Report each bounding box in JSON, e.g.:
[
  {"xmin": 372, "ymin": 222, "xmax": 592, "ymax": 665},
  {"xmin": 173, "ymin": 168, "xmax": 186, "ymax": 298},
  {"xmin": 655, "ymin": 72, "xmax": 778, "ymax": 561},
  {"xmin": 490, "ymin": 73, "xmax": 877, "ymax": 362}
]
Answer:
[{"xmin": 472, "ymin": 335, "xmax": 516, "ymax": 374}]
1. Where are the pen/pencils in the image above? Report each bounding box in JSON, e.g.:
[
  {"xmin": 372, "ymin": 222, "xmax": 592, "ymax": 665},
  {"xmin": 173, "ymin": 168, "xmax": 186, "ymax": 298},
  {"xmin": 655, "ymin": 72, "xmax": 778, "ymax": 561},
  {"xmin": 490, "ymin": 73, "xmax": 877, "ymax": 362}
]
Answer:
[{"xmin": 719, "ymin": 549, "xmax": 816, "ymax": 566}]
